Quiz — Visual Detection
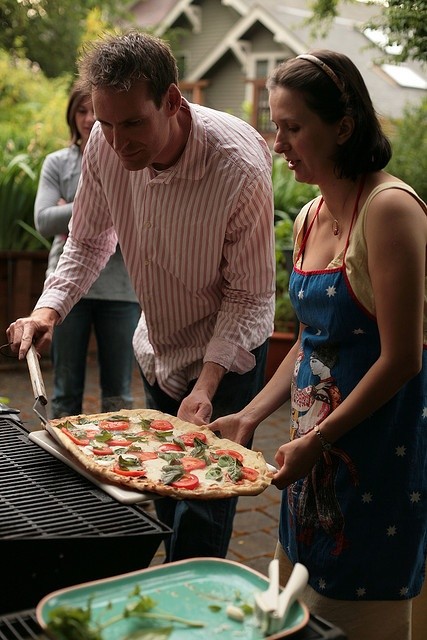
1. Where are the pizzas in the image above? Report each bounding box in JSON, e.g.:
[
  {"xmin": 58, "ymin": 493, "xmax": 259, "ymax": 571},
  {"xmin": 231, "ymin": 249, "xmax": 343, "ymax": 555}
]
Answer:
[{"xmin": 40, "ymin": 407, "xmax": 273, "ymax": 499}]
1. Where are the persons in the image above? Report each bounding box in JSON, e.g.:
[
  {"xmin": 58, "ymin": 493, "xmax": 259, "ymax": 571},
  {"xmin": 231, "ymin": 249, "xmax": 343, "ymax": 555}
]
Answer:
[
  {"xmin": 6, "ymin": 28, "xmax": 277, "ymax": 559},
  {"xmin": 200, "ymin": 52, "xmax": 427, "ymax": 639},
  {"xmin": 34, "ymin": 83, "xmax": 154, "ymax": 413}
]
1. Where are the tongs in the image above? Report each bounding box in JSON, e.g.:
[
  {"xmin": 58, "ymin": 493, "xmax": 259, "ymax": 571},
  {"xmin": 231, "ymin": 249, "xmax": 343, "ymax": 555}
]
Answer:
[{"xmin": 255, "ymin": 556, "xmax": 308, "ymax": 635}]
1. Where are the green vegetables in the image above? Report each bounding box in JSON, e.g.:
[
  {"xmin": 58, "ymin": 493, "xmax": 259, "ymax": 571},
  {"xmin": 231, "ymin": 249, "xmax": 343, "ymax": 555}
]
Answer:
[{"xmin": 45, "ymin": 586, "xmax": 204, "ymax": 639}]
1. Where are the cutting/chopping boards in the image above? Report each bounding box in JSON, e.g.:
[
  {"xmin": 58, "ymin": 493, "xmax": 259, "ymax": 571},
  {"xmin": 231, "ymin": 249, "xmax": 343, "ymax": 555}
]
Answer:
[{"xmin": 29, "ymin": 430, "xmax": 278, "ymax": 505}]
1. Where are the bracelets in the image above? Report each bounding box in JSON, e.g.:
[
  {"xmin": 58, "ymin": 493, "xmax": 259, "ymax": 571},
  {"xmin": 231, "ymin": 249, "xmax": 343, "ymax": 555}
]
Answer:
[{"xmin": 310, "ymin": 425, "xmax": 343, "ymax": 459}]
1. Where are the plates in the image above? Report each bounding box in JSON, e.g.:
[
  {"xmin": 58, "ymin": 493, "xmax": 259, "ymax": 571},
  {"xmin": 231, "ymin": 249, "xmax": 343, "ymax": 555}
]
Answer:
[{"xmin": 35, "ymin": 556, "xmax": 310, "ymax": 639}]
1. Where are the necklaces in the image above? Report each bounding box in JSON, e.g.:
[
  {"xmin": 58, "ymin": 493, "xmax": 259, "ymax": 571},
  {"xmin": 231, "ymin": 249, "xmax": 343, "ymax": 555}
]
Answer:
[{"xmin": 319, "ymin": 195, "xmax": 359, "ymax": 237}]
